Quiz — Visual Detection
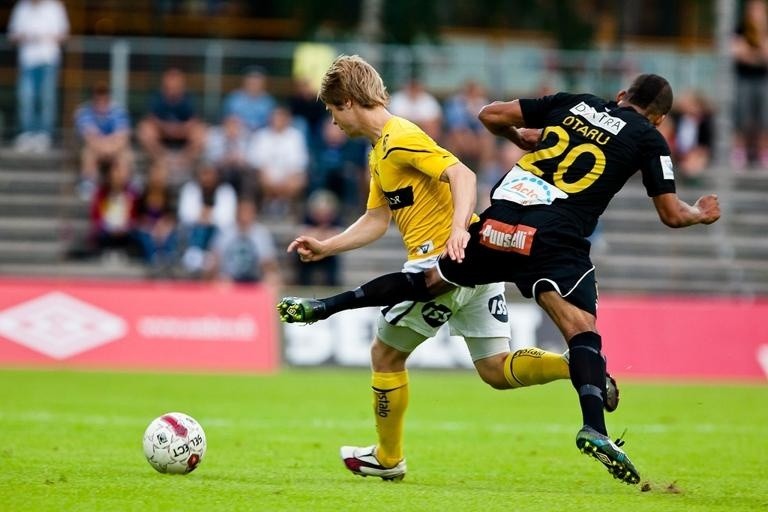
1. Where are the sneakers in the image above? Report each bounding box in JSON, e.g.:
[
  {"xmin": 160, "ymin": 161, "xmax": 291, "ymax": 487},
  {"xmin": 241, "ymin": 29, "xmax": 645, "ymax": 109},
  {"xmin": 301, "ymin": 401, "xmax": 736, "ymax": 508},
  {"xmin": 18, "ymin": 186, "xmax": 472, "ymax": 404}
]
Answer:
[
  {"xmin": 276, "ymin": 297, "xmax": 326, "ymax": 325},
  {"xmin": 574, "ymin": 423, "xmax": 641, "ymax": 485},
  {"xmin": 564, "ymin": 349, "xmax": 619, "ymax": 413},
  {"xmin": 341, "ymin": 445, "xmax": 406, "ymax": 481}
]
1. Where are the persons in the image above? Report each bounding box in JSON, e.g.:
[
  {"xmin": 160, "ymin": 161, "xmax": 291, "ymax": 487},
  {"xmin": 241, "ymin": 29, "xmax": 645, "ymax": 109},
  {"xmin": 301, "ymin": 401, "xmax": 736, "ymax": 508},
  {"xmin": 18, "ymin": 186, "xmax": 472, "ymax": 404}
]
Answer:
[
  {"xmin": 6, "ymin": 1, "xmax": 72, "ymax": 154},
  {"xmin": 730, "ymin": 2, "xmax": 768, "ymax": 172},
  {"xmin": 275, "ymin": 69, "xmax": 723, "ymax": 486},
  {"xmin": 286, "ymin": 54, "xmax": 624, "ymax": 483},
  {"xmin": 63, "ymin": 13, "xmax": 717, "ymax": 289}
]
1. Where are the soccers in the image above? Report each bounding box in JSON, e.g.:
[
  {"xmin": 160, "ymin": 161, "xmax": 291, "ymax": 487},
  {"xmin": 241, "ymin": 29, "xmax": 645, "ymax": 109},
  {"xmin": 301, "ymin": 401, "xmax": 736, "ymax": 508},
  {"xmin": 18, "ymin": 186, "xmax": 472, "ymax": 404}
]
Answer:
[{"xmin": 142, "ymin": 411, "xmax": 206, "ymax": 474}]
